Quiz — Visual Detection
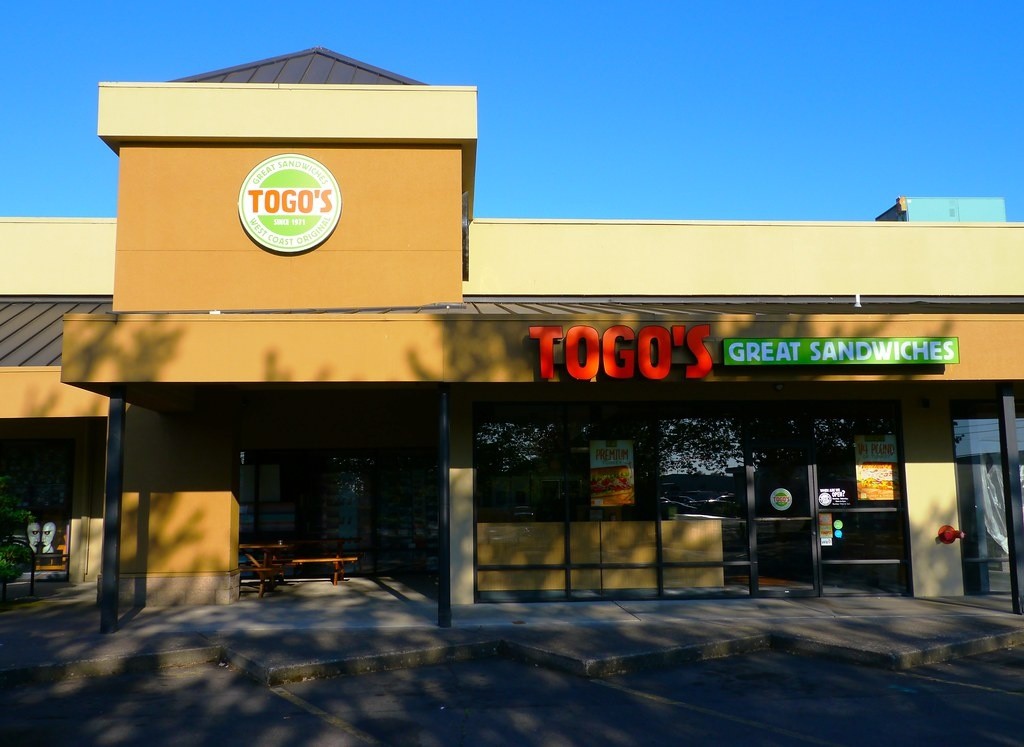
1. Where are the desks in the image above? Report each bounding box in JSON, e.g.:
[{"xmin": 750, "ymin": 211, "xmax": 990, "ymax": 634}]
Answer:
[
  {"xmin": 241, "ymin": 568, "xmax": 272, "ymax": 597},
  {"xmin": 237, "ymin": 544, "xmax": 291, "ymax": 584},
  {"xmin": 258, "ymin": 538, "xmax": 347, "ymax": 584}
]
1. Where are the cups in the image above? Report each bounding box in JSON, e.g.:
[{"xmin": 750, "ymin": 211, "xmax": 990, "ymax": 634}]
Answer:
[{"xmin": 610, "ymin": 515, "xmax": 616, "ymax": 520}]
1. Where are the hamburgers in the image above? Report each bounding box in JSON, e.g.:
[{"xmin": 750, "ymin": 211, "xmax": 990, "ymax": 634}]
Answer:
[{"xmin": 589, "ymin": 466, "xmax": 632, "ymax": 498}]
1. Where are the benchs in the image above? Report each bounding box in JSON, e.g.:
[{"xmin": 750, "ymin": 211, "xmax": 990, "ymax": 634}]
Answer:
[{"xmin": 249, "ymin": 557, "xmax": 357, "ymax": 586}]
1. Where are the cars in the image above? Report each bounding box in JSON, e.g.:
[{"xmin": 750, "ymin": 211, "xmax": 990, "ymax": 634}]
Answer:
[{"xmin": 502, "ymin": 485, "xmax": 746, "ymax": 524}]
